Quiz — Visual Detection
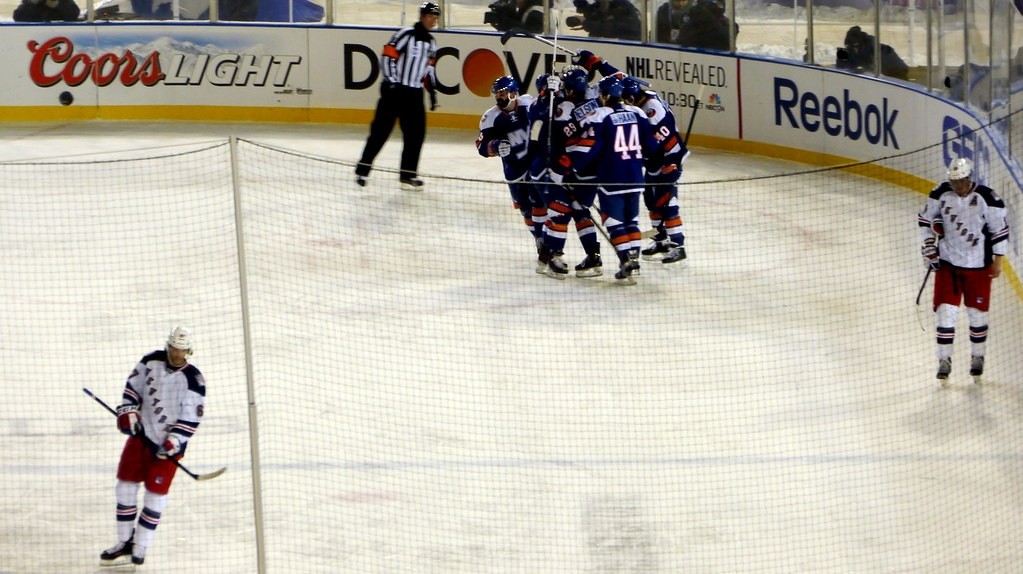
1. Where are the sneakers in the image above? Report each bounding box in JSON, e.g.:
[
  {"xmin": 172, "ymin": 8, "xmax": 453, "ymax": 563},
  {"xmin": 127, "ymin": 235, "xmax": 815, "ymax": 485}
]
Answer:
[
  {"xmin": 662, "ymin": 242, "xmax": 689, "ymax": 271},
  {"xmin": 642, "ymin": 231, "xmax": 669, "ymax": 260},
  {"xmin": 529, "ymin": 229, "xmax": 567, "ymax": 274},
  {"xmin": 969, "ymin": 354, "xmax": 984, "ymax": 383},
  {"xmin": 629, "ymin": 250, "xmax": 640, "ymax": 275},
  {"xmin": 131, "ymin": 542, "xmax": 146, "ymax": 567},
  {"xmin": 937, "ymin": 358, "xmax": 951, "ymax": 388},
  {"xmin": 100, "ymin": 527, "xmax": 134, "ymax": 565},
  {"xmin": 543, "ymin": 248, "xmax": 569, "ymax": 280},
  {"xmin": 615, "ymin": 248, "xmax": 637, "ymax": 285},
  {"xmin": 575, "ymin": 241, "xmax": 604, "ymax": 277}
]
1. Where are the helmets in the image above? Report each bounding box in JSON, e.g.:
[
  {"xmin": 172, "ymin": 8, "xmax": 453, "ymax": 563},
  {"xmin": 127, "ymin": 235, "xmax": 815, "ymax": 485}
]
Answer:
[
  {"xmin": 536, "ymin": 73, "xmax": 551, "ymax": 89},
  {"xmin": 356, "ymin": 175, "xmax": 368, "ymax": 187},
  {"xmin": 598, "ymin": 76, "xmax": 625, "ymax": 98},
  {"xmin": 165, "ymin": 325, "xmax": 193, "ymax": 368},
  {"xmin": 399, "ymin": 177, "xmax": 424, "ymax": 191},
  {"xmin": 946, "ymin": 157, "xmax": 973, "ymax": 181},
  {"xmin": 621, "ymin": 77, "xmax": 642, "ymax": 99},
  {"xmin": 492, "ymin": 75, "xmax": 519, "ymax": 93},
  {"xmin": 560, "ymin": 65, "xmax": 589, "ymax": 93},
  {"xmin": 420, "ymin": 1, "xmax": 440, "ymax": 15}
]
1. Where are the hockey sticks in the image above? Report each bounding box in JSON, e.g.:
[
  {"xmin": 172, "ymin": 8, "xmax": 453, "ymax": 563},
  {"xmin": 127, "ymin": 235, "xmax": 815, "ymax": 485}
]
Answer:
[
  {"xmin": 591, "ymin": 202, "xmax": 601, "ymax": 215},
  {"xmin": 499, "ymin": 26, "xmax": 653, "ymax": 89},
  {"xmin": 531, "ymin": 5, "xmax": 559, "ymax": 195},
  {"xmin": 640, "ymin": 83, "xmax": 706, "ymax": 240},
  {"xmin": 80, "ymin": 386, "xmax": 229, "ymax": 483},
  {"xmin": 563, "ymin": 182, "xmax": 619, "ymax": 253},
  {"xmin": 914, "ymin": 238, "xmax": 941, "ymax": 332}
]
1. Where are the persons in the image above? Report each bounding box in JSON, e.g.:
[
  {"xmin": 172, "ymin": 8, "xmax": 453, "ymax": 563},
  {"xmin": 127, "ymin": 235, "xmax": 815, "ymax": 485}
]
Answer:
[
  {"xmin": 476, "ymin": 49, "xmax": 691, "ymax": 279},
  {"xmin": 836, "ymin": 26, "xmax": 910, "ymax": 81},
  {"xmin": 655, "ymin": 0, "xmax": 739, "ymax": 51},
  {"xmin": 98, "ymin": 326, "xmax": 206, "ymax": 565},
  {"xmin": 491, "ymin": 0, "xmax": 559, "ymax": 35},
  {"xmin": 13, "ymin": 0, "xmax": 80, "ymax": 22},
  {"xmin": 354, "ymin": 2, "xmax": 442, "ymax": 187},
  {"xmin": 918, "ymin": 158, "xmax": 1010, "ymax": 379},
  {"xmin": 573, "ymin": 0, "xmax": 642, "ymax": 41}
]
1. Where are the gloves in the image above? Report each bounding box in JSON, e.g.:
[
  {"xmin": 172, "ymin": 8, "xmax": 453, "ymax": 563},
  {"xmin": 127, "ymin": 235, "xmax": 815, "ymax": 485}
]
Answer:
[
  {"xmin": 430, "ymin": 92, "xmax": 436, "ymax": 111},
  {"xmin": 547, "ymin": 155, "xmax": 575, "ymax": 185},
  {"xmin": 661, "ymin": 163, "xmax": 682, "ymax": 183},
  {"xmin": 547, "ymin": 75, "xmax": 561, "ymax": 92},
  {"xmin": 610, "ymin": 72, "xmax": 629, "ymax": 82},
  {"xmin": 571, "ymin": 48, "xmax": 601, "ymax": 73},
  {"xmin": 154, "ymin": 434, "xmax": 185, "ymax": 463},
  {"xmin": 921, "ymin": 238, "xmax": 941, "ymax": 272},
  {"xmin": 491, "ymin": 138, "xmax": 512, "ymax": 158},
  {"xmin": 117, "ymin": 403, "xmax": 142, "ymax": 438}
]
